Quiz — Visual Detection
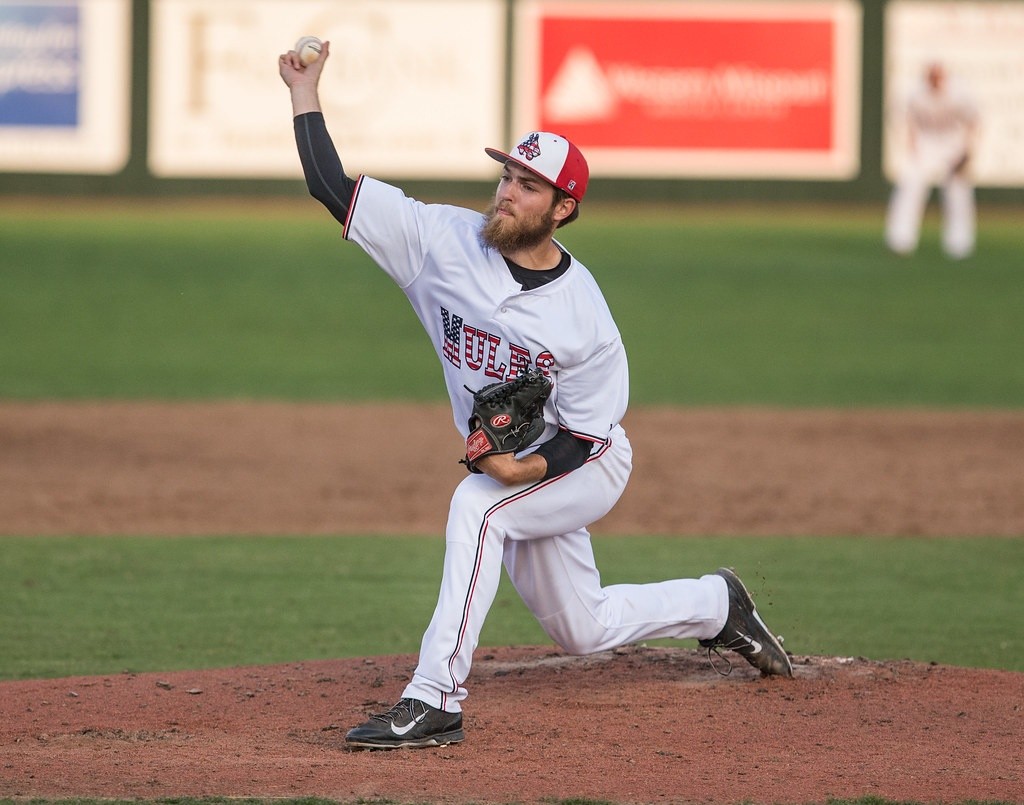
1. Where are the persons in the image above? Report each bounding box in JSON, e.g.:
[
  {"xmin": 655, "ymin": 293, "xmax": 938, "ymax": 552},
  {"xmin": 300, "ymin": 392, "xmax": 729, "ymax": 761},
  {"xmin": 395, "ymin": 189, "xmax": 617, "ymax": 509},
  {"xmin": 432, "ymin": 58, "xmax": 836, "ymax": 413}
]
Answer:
[
  {"xmin": 881, "ymin": 57, "xmax": 977, "ymax": 258},
  {"xmin": 279, "ymin": 40, "xmax": 795, "ymax": 747}
]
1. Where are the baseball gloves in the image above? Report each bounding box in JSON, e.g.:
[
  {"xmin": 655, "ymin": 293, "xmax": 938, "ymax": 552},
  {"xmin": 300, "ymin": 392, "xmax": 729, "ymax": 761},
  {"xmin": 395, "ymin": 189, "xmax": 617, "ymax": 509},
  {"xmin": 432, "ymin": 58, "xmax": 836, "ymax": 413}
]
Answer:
[{"xmin": 464, "ymin": 373, "xmax": 553, "ymax": 473}]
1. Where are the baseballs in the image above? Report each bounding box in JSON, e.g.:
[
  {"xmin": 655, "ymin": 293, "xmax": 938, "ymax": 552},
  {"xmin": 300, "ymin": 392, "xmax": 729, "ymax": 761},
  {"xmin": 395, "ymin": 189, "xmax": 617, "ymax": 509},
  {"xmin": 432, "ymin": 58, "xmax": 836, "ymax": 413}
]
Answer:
[{"xmin": 295, "ymin": 36, "xmax": 322, "ymax": 67}]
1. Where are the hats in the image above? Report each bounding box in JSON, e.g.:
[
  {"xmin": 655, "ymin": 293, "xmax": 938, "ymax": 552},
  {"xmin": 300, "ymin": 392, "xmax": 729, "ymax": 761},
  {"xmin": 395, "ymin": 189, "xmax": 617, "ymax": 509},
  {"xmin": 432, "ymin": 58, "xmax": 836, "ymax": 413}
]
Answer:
[{"xmin": 485, "ymin": 132, "xmax": 589, "ymax": 203}]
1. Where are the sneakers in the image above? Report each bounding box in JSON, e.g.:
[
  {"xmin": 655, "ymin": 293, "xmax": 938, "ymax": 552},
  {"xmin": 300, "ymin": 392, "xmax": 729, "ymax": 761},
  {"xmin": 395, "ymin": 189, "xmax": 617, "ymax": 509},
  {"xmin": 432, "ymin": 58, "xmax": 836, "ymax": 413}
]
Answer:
[
  {"xmin": 698, "ymin": 567, "xmax": 794, "ymax": 678},
  {"xmin": 345, "ymin": 697, "xmax": 465, "ymax": 750}
]
